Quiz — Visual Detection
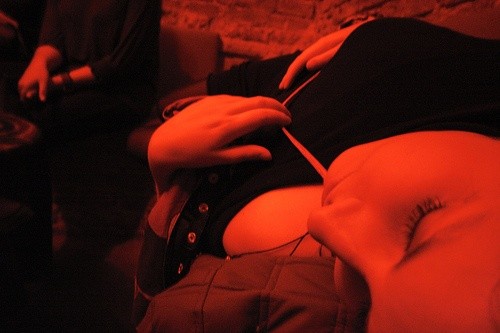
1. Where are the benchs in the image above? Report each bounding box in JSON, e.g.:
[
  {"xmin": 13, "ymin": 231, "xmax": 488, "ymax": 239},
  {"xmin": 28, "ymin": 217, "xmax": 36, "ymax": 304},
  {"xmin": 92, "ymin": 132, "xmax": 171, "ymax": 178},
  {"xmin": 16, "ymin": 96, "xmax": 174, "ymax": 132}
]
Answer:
[{"xmin": 54, "ymin": 28, "xmax": 221, "ymax": 238}]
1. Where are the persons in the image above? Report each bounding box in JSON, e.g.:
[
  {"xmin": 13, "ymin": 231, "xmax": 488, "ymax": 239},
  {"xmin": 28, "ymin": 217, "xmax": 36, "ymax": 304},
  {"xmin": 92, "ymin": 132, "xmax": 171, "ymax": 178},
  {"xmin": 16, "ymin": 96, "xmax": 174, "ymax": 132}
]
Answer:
[
  {"xmin": 0, "ymin": 0, "xmax": 163, "ymax": 148},
  {"xmin": 123, "ymin": 13, "xmax": 500, "ymax": 333}
]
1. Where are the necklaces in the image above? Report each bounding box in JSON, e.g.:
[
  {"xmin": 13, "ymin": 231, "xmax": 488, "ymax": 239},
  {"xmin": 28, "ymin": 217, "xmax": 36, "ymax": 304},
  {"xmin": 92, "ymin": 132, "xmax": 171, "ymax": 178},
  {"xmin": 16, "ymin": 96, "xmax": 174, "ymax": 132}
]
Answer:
[{"xmin": 219, "ymin": 231, "xmax": 311, "ymax": 262}]
